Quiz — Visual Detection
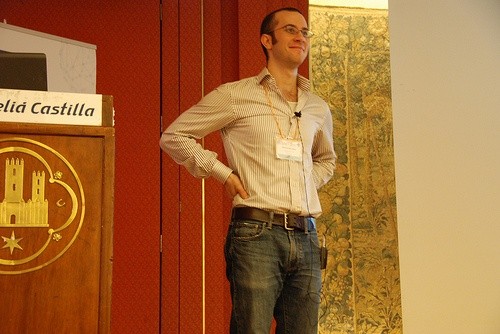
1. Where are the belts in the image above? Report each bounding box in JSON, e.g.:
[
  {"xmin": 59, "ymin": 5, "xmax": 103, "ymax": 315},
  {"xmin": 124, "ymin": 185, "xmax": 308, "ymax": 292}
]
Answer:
[{"xmin": 230, "ymin": 206, "xmax": 317, "ymax": 233}]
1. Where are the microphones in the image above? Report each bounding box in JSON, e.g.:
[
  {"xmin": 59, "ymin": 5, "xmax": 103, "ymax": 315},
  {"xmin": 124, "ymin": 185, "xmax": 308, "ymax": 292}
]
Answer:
[{"xmin": 294, "ymin": 111, "xmax": 302, "ymax": 117}]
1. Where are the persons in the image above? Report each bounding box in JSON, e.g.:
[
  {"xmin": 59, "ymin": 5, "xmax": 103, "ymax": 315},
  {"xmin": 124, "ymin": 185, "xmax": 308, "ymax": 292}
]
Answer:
[{"xmin": 160, "ymin": 6, "xmax": 340, "ymax": 334}]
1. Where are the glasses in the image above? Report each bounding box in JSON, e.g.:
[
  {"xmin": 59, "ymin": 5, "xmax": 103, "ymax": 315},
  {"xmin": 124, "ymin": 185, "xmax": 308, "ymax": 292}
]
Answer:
[{"xmin": 269, "ymin": 25, "xmax": 314, "ymax": 38}]
